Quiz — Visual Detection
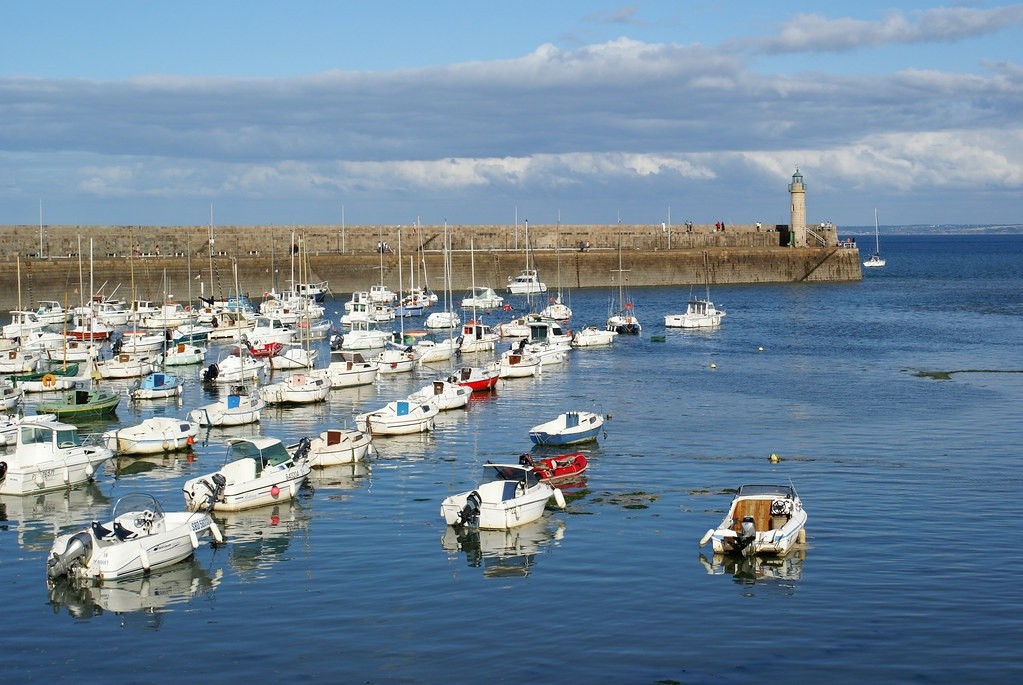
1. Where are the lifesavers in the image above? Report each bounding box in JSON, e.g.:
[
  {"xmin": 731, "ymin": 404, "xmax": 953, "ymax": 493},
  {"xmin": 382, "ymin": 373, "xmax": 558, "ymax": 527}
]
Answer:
[
  {"xmin": 625, "ymin": 303, "xmax": 632, "ymax": 309},
  {"xmin": 262, "ymin": 291, "xmax": 269, "ymax": 297},
  {"xmin": 42, "ymin": 374, "xmax": 56, "ymax": 387},
  {"xmin": 185, "ymin": 306, "xmax": 191, "ymax": 311},
  {"xmin": 404, "ymin": 288, "xmax": 411, "ymax": 294}
]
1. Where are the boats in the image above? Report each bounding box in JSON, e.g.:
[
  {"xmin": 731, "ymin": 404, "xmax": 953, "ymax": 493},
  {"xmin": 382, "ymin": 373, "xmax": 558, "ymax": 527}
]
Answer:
[
  {"xmin": 496, "ymin": 451, "xmax": 589, "ymax": 486},
  {"xmin": 439, "ymin": 464, "xmax": 567, "ymax": 530},
  {"xmin": 663, "ymin": 282, "xmax": 727, "ymax": 329},
  {"xmin": 529, "ymin": 410, "xmax": 604, "ymax": 446},
  {"xmin": 700, "ymin": 475, "xmax": 808, "ymax": 555}
]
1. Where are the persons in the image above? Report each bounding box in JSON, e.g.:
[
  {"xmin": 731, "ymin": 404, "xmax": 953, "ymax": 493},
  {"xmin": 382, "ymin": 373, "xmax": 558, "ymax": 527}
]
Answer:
[
  {"xmin": 586, "ymin": 241, "xmax": 590, "ymax": 251},
  {"xmin": 852, "ymin": 238, "xmax": 855, "ymax": 248},
  {"xmin": 820, "ymin": 221, "xmax": 833, "ymax": 231},
  {"xmin": 755, "ymin": 221, "xmax": 761, "ymax": 232},
  {"xmin": 579, "ymin": 241, "xmax": 585, "ymax": 252},
  {"xmin": 841, "ymin": 239, "xmax": 844, "ymax": 247},
  {"xmin": 378, "ymin": 241, "xmax": 393, "ymax": 254},
  {"xmin": 661, "ymin": 221, "xmax": 665, "ymax": 232},
  {"xmin": 721, "ymin": 222, "xmax": 725, "ymax": 231},
  {"xmin": 846, "ymin": 238, "xmax": 851, "ymax": 245},
  {"xmin": 716, "ymin": 222, "xmax": 720, "ymax": 231}
]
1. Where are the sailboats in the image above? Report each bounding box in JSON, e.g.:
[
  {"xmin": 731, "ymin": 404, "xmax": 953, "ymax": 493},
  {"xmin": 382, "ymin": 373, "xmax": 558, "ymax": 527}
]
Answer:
[
  {"xmin": 862, "ymin": 208, "xmax": 886, "ymax": 267},
  {"xmin": 0, "ymin": 216, "xmax": 643, "ymax": 588}
]
